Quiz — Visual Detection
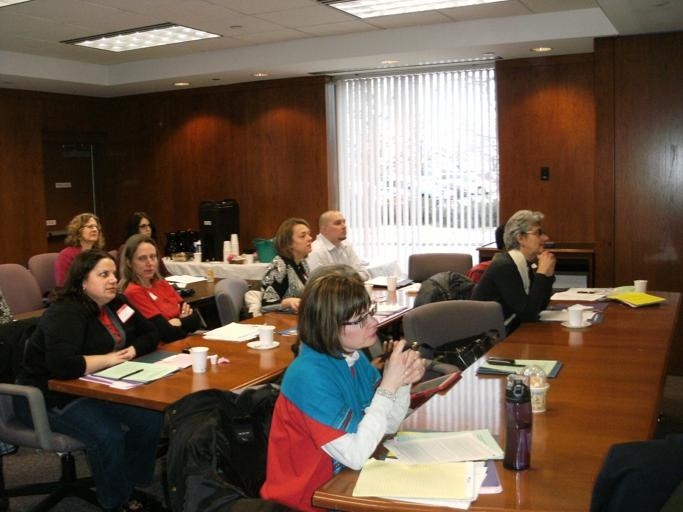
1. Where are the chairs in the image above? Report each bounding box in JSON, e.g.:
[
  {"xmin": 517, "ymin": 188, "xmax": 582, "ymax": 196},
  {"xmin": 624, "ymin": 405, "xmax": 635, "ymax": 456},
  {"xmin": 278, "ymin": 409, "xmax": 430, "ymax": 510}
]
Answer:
[
  {"xmin": 0, "ymin": 248, "xmax": 119, "ymax": 318},
  {"xmin": 215, "ymin": 278, "xmax": 260, "ymax": 327},
  {"xmin": 405, "ymin": 252, "xmax": 474, "ymax": 275},
  {"xmin": 401, "ymin": 300, "xmax": 505, "ymax": 376},
  {"xmin": 169, "ymin": 385, "xmax": 325, "ymax": 512}
]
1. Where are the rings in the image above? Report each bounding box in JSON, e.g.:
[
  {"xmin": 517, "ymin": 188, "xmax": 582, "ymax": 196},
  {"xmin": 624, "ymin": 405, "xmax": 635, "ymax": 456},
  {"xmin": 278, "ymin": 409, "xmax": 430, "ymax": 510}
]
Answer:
[{"xmin": 180, "ymin": 310, "xmax": 186, "ymax": 314}]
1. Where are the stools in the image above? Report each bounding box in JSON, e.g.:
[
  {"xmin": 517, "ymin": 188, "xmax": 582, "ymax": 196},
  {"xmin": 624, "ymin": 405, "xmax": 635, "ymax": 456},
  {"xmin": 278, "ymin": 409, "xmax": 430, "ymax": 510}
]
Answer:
[{"xmin": 0, "ymin": 380, "xmax": 90, "ymax": 509}]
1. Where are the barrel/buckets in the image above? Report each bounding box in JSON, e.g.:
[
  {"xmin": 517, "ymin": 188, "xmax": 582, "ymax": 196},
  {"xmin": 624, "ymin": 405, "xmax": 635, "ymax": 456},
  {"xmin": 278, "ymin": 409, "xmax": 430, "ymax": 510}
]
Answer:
[{"xmin": 255, "ymin": 235, "xmax": 281, "ymax": 264}]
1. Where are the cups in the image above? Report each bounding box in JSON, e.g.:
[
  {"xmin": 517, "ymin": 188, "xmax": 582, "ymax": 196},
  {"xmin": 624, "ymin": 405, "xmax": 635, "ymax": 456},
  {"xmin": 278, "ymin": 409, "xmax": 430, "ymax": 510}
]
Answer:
[
  {"xmin": 256, "ymin": 325, "xmax": 275, "ymax": 346},
  {"xmin": 192, "ymin": 234, "xmax": 254, "ymax": 263},
  {"xmin": 363, "ymin": 285, "xmax": 373, "ymax": 295},
  {"xmin": 633, "ymin": 279, "xmax": 648, "ymax": 297},
  {"xmin": 257, "ymin": 349, "xmax": 276, "ymax": 370},
  {"xmin": 188, "ymin": 346, "xmax": 209, "ymax": 374},
  {"xmin": 386, "ymin": 291, "xmax": 396, "ymax": 305},
  {"xmin": 567, "ymin": 307, "xmax": 584, "ymax": 325},
  {"xmin": 385, "ymin": 275, "xmax": 396, "ymax": 291},
  {"xmin": 522, "ymin": 363, "xmax": 550, "ymax": 414},
  {"xmin": 567, "ymin": 330, "xmax": 584, "ymax": 347},
  {"xmin": 190, "ymin": 372, "xmax": 208, "ymax": 393}
]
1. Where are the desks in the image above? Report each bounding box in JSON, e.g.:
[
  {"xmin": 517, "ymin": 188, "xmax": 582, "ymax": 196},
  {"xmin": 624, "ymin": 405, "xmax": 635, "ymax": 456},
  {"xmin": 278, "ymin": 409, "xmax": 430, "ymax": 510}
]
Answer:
[
  {"xmin": 161, "ymin": 255, "xmax": 287, "ymax": 279},
  {"xmin": 476, "ymin": 242, "xmax": 598, "ymax": 275},
  {"xmin": 314, "ymin": 288, "xmax": 683, "ymax": 510},
  {"xmin": 49, "ymin": 278, "xmax": 425, "ymax": 510}
]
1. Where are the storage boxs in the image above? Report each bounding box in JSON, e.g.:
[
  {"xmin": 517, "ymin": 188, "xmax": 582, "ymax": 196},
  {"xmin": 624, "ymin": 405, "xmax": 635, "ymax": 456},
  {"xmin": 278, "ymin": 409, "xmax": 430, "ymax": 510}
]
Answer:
[{"xmin": 253, "ymin": 237, "xmax": 280, "ymax": 262}]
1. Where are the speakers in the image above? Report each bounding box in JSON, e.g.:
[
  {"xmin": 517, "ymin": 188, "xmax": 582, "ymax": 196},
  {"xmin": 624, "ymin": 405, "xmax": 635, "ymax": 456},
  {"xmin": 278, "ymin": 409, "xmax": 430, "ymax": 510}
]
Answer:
[{"xmin": 199, "ymin": 198, "xmax": 239, "ymax": 262}]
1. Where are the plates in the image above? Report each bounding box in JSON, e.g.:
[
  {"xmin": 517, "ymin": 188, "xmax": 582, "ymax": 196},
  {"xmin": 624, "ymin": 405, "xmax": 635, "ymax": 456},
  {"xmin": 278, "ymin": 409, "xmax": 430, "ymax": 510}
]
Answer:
[
  {"xmin": 245, "ymin": 340, "xmax": 279, "ymax": 351},
  {"xmin": 560, "ymin": 321, "xmax": 592, "ymax": 329}
]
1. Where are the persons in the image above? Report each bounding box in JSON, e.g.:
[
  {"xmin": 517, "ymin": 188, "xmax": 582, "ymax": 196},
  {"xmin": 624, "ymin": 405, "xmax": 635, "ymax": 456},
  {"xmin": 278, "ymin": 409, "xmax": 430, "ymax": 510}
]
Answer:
[
  {"xmin": 469, "ymin": 209, "xmax": 558, "ymax": 323},
  {"xmin": 302, "ymin": 209, "xmax": 372, "ymax": 283},
  {"xmin": 11, "ymin": 248, "xmax": 169, "ymax": 512},
  {"xmin": 52, "ymin": 212, "xmax": 107, "ymax": 290},
  {"xmin": 258, "ymin": 216, "xmax": 313, "ymax": 314},
  {"xmin": 115, "ymin": 233, "xmax": 200, "ymax": 348},
  {"xmin": 259, "ymin": 263, "xmax": 428, "ymax": 512},
  {"xmin": 123, "ymin": 208, "xmax": 172, "ymax": 277},
  {"xmin": 464, "ymin": 222, "xmax": 510, "ymax": 284}
]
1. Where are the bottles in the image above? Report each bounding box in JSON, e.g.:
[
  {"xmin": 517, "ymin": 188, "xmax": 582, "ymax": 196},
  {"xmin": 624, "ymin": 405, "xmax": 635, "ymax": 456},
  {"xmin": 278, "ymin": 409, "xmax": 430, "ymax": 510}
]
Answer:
[
  {"xmin": 502, "ymin": 372, "xmax": 531, "ymax": 471},
  {"xmin": 503, "ymin": 468, "xmax": 532, "ymax": 510}
]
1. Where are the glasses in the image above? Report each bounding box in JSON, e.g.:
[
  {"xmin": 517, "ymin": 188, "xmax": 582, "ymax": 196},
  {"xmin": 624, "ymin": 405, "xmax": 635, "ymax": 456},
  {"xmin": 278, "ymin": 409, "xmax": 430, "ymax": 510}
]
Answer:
[
  {"xmin": 340, "ymin": 301, "xmax": 377, "ymax": 325},
  {"xmin": 511, "ymin": 229, "xmax": 542, "ymax": 237}
]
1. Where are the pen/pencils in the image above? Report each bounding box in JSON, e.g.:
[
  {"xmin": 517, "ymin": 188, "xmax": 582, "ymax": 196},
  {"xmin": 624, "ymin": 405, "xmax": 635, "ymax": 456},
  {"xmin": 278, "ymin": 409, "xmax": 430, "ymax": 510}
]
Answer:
[
  {"xmin": 378, "ymin": 341, "xmax": 418, "ymax": 363},
  {"xmin": 503, "ymin": 364, "xmax": 528, "ymax": 367},
  {"xmin": 119, "ymin": 369, "xmax": 143, "ymax": 380},
  {"xmin": 577, "ymin": 291, "xmax": 595, "ymax": 294}
]
